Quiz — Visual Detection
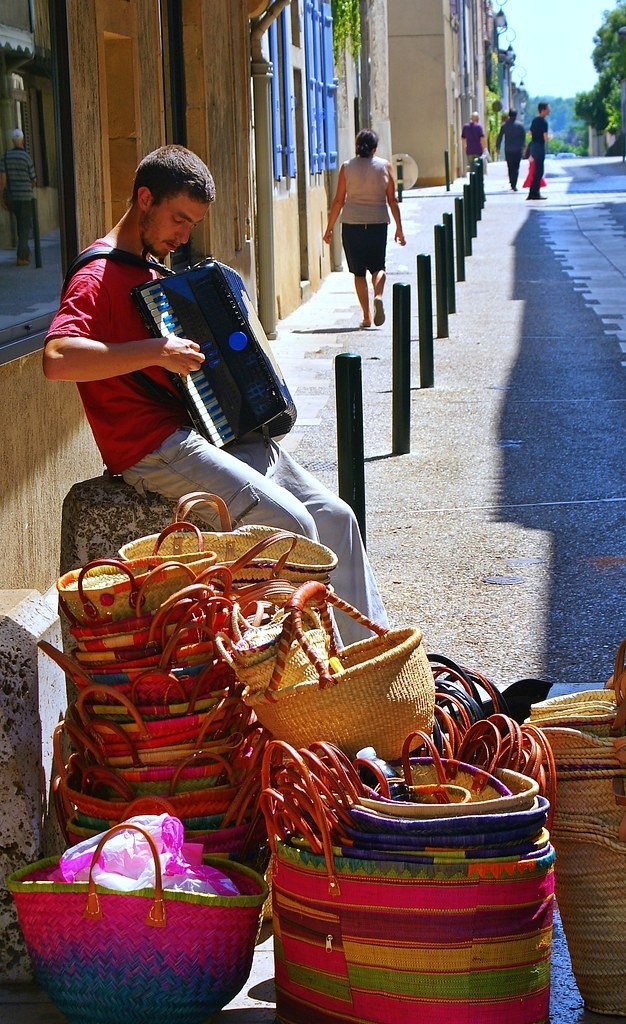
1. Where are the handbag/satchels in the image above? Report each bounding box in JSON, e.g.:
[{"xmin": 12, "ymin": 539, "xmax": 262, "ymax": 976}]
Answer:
[
  {"xmin": 4, "ymin": 492, "xmax": 626, "ymax": 1024},
  {"xmin": 478, "ymin": 148, "xmax": 492, "ymax": 176},
  {"xmin": 522, "ymin": 155, "xmax": 547, "ymax": 188}
]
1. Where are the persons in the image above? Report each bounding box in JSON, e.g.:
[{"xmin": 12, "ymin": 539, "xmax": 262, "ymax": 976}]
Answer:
[
  {"xmin": 523, "ymin": 102, "xmax": 551, "ymax": 200},
  {"xmin": 0, "ymin": 128, "xmax": 38, "ymax": 265},
  {"xmin": 323, "ymin": 129, "xmax": 407, "ymax": 327},
  {"xmin": 42, "ymin": 144, "xmax": 390, "ymax": 650},
  {"xmin": 461, "ymin": 111, "xmax": 492, "ymax": 175},
  {"xmin": 495, "ymin": 108, "xmax": 526, "ymax": 191}
]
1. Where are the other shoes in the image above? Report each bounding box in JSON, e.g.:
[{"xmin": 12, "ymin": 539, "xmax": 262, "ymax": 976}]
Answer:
[
  {"xmin": 372, "ymin": 297, "xmax": 385, "ymax": 326},
  {"xmin": 360, "ymin": 320, "xmax": 370, "ymax": 327},
  {"xmin": 532, "ymin": 196, "xmax": 547, "ymax": 200}
]
